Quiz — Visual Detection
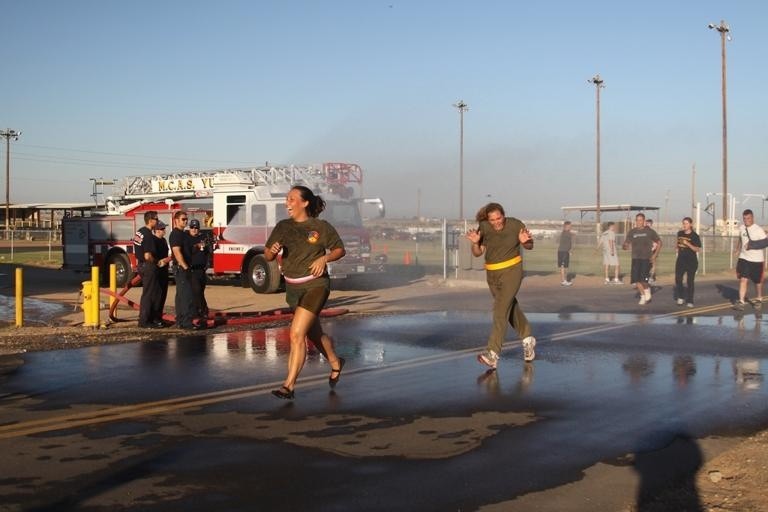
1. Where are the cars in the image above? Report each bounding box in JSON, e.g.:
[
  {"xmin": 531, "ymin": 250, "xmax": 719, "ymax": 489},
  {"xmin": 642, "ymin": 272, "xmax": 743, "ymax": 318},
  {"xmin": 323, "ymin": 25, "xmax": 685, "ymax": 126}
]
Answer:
[{"xmin": 377, "ymin": 223, "xmax": 583, "ymax": 243}]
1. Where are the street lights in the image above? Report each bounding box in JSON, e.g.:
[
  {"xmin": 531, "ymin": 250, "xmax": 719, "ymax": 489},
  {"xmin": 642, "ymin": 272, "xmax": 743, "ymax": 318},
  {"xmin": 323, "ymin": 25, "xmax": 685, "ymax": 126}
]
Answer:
[
  {"xmin": 451, "ymin": 101, "xmax": 471, "ymax": 223},
  {"xmin": 586, "ymin": 72, "xmax": 605, "ymax": 237},
  {"xmin": 707, "ymin": 18, "xmax": 732, "ymax": 230},
  {"xmin": 0, "ymin": 125, "xmax": 25, "ymax": 240}
]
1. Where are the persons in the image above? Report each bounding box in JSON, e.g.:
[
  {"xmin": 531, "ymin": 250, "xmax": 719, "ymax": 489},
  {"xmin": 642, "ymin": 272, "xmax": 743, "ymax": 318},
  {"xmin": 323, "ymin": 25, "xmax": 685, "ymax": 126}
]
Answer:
[
  {"xmin": 169, "ymin": 211, "xmax": 199, "ymax": 330},
  {"xmin": 264, "ymin": 186, "xmax": 346, "ymax": 404},
  {"xmin": 464, "ymin": 203, "xmax": 536, "ymax": 369},
  {"xmin": 594, "ymin": 222, "xmax": 624, "ymax": 286},
  {"xmin": 189, "ymin": 219, "xmax": 211, "ymax": 316},
  {"xmin": 134, "ymin": 211, "xmax": 162, "ymax": 329},
  {"xmin": 558, "ymin": 221, "xmax": 574, "ymax": 287},
  {"xmin": 646, "ymin": 218, "xmax": 655, "ymax": 286},
  {"xmin": 675, "ymin": 217, "xmax": 701, "ymax": 307},
  {"xmin": 732, "ymin": 209, "xmax": 768, "ymax": 312},
  {"xmin": 155, "ymin": 219, "xmax": 169, "ymax": 326},
  {"xmin": 623, "ymin": 213, "xmax": 662, "ymax": 304}
]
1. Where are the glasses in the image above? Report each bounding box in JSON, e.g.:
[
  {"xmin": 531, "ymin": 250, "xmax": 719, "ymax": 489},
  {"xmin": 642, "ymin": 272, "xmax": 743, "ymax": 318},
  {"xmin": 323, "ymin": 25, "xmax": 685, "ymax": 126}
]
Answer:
[
  {"xmin": 190, "ymin": 227, "xmax": 198, "ymax": 229},
  {"xmin": 177, "ymin": 217, "xmax": 188, "ymax": 221}
]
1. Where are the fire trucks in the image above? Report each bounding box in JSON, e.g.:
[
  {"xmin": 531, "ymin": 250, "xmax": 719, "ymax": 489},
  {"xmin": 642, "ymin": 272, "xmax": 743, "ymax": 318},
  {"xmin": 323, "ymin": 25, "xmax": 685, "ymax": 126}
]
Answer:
[
  {"xmin": 143, "ymin": 320, "xmax": 387, "ymax": 366},
  {"xmin": 60, "ymin": 159, "xmax": 388, "ymax": 295}
]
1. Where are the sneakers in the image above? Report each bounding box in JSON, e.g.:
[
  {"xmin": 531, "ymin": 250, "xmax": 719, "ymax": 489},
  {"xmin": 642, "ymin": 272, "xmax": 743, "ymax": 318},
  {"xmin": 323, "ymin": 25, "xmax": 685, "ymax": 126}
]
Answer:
[
  {"xmin": 272, "ymin": 387, "xmax": 295, "ymax": 400},
  {"xmin": 752, "ymin": 300, "xmax": 762, "ymax": 310},
  {"xmin": 677, "ymin": 297, "xmax": 683, "ymax": 305},
  {"xmin": 477, "ymin": 349, "xmax": 499, "ymax": 370},
  {"xmin": 687, "ymin": 303, "xmax": 693, "ymax": 308},
  {"xmin": 523, "ymin": 336, "xmax": 537, "ymax": 360},
  {"xmin": 638, "ymin": 295, "xmax": 645, "ymax": 305},
  {"xmin": 643, "ymin": 287, "xmax": 652, "ymax": 301},
  {"xmin": 560, "ymin": 280, "xmax": 572, "ymax": 287},
  {"xmin": 733, "ymin": 300, "xmax": 745, "ymax": 311},
  {"xmin": 604, "ymin": 280, "xmax": 623, "ymax": 285},
  {"xmin": 329, "ymin": 357, "xmax": 345, "ymax": 387}
]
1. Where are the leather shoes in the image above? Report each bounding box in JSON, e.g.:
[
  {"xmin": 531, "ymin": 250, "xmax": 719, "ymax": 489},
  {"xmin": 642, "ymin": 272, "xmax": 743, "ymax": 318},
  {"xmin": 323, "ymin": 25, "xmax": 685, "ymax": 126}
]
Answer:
[
  {"xmin": 178, "ymin": 324, "xmax": 201, "ymax": 330},
  {"xmin": 138, "ymin": 320, "xmax": 165, "ymax": 328}
]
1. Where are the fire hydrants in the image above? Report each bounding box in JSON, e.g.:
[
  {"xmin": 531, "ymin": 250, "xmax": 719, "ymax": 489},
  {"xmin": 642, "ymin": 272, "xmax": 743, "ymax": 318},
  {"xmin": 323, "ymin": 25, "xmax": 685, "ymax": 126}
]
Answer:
[{"xmin": 73, "ymin": 280, "xmax": 96, "ymax": 327}]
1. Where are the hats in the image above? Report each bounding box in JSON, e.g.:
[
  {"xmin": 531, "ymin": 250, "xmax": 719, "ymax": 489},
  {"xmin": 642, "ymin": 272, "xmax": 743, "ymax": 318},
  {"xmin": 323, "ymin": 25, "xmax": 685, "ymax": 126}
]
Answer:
[
  {"xmin": 189, "ymin": 220, "xmax": 200, "ymax": 227},
  {"xmin": 155, "ymin": 221, "xmax": 168, "ymax": 230}
]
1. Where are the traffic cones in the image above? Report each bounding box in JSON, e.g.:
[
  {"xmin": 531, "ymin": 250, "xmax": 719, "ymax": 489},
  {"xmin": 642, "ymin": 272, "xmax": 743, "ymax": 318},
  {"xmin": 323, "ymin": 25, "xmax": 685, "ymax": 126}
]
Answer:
[
  {"xmin": 371, "ymin": 240, "xmax": 389, "ymax": 255},
  {"xmin": 397, "ymin": 252, "xmax": 414, "ymax": 267}
]
